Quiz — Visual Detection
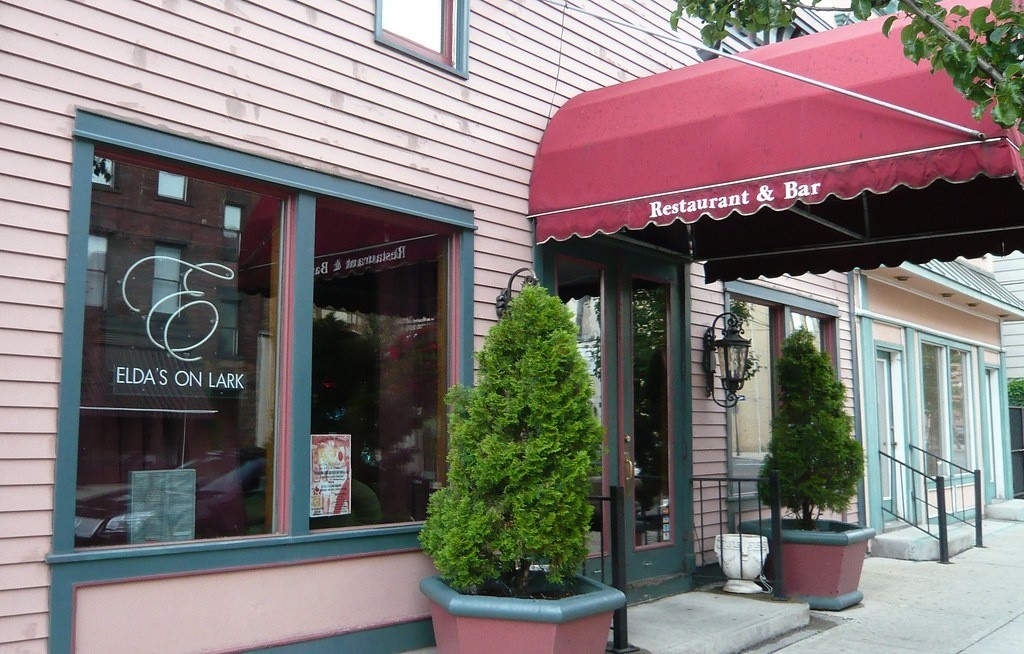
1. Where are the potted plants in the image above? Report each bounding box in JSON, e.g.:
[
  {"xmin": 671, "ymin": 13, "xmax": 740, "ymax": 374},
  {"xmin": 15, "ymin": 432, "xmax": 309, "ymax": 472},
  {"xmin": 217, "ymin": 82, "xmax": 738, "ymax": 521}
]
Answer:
[
  {"xmin": 418, "ymin": 287, "xmax": 627, "ymax": 653},
  {"xmin": 754, "ymin": 324, "xmax": 876, "ymax": 611}
]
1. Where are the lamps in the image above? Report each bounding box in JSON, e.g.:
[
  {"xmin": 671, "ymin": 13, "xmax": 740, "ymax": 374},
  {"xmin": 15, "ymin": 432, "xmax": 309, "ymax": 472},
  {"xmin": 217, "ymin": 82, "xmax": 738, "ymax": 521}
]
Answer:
[{"xmin": 703, "ymin": 312, "xmax": 751, "ymax": 408}]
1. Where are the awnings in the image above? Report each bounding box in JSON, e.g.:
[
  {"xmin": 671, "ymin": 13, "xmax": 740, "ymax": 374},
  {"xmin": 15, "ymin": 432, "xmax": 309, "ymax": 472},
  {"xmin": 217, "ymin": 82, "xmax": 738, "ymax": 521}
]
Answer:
[
  {"xmin": 79, "ymin": 344, "xmax": 217, "ymax": 419},
  {"xmin": 529, "ymin": 0, "xmax": 1023, "ymax": 301}
]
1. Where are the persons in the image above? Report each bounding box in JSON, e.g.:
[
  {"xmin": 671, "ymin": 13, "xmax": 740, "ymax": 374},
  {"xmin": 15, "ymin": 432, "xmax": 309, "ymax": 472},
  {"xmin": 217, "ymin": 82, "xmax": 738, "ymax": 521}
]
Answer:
[
  {"xmin": 238, "ymin": 447, "xmax": 266, "ymax": 535},
  {"xmin": 310, "ymin": 479, "xmax": 381, "ymax": 531}
]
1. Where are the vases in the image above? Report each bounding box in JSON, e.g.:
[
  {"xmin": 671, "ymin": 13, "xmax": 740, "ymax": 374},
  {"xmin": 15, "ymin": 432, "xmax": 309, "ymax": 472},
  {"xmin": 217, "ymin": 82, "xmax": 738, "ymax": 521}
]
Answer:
[{"xmin": 714, "ymin": 533, "xmax": 770, "ymax": 594}]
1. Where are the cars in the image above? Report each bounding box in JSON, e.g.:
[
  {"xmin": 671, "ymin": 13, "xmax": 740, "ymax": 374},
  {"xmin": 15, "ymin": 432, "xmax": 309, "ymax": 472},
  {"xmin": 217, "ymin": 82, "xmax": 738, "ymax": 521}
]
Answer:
[{"xmin": 74, "ymin": 438, "xmax": 416, "ymax": 548}]
